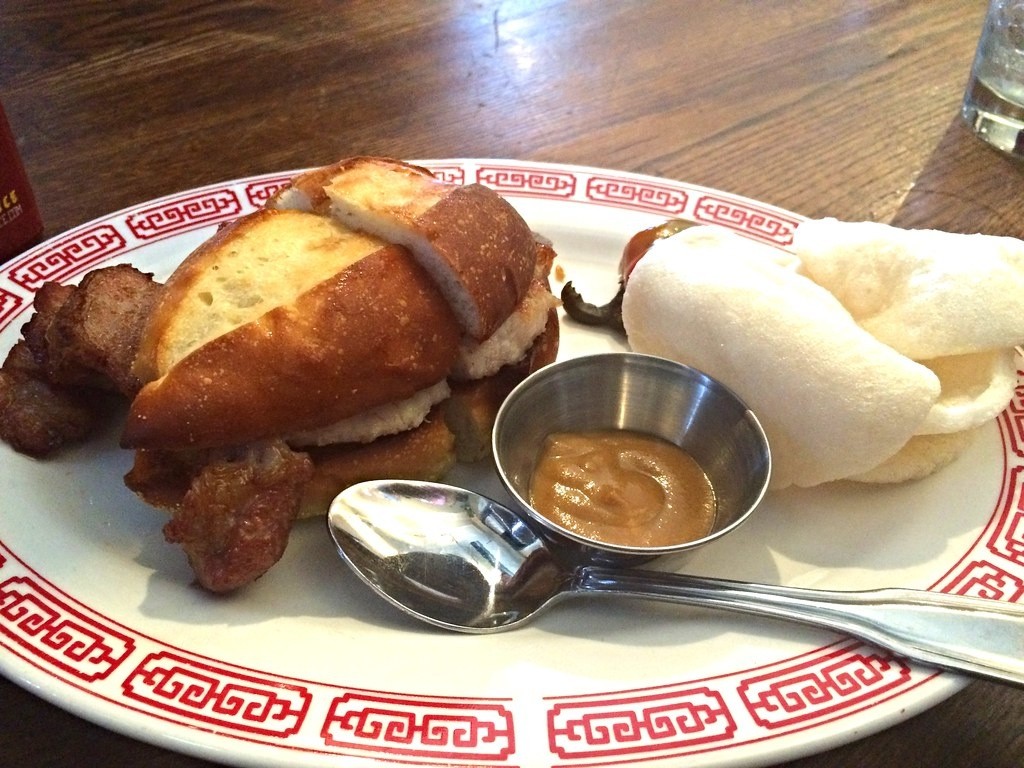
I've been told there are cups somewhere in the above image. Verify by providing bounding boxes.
[958,0,1024,160]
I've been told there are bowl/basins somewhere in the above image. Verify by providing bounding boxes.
[493,353,774,568]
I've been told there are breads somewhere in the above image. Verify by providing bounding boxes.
[116,158,562,521]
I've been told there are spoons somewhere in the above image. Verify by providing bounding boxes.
[326,478,1024,687]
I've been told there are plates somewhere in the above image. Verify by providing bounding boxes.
[0,153,1024,768]
